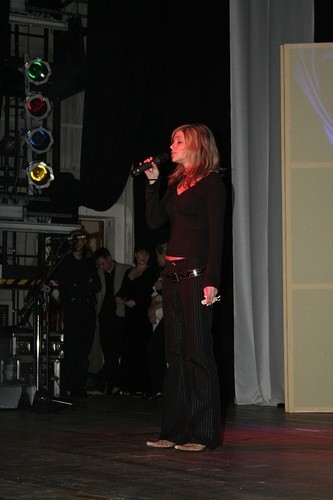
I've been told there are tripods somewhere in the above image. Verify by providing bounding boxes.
[19,239,90,410]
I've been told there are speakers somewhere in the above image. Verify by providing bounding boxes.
[0,384,32,409]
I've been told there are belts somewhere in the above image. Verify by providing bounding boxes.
[161,267,206,283]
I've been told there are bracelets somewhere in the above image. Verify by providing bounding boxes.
[148,178,158,181]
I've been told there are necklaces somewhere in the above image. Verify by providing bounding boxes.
[132,268,138,274]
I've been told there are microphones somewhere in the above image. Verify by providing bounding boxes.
[132,153,169,176]
[63,235,87,241]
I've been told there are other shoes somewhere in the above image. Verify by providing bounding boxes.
[103,387,164,401]
[174,443,210,452]
[145,439,176,448]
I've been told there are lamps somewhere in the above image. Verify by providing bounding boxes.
[24,58,56,190]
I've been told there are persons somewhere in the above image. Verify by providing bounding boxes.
[146,240,168,401]
[112,243,158,400]
[92,247,132,396]
[51,229,101,397]
[138,122,226,452]
